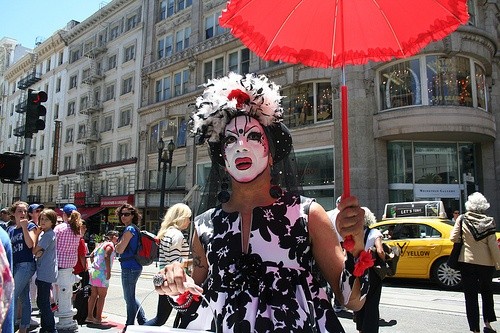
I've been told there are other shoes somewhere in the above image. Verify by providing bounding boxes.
[85,317,95,322]
[334,309,354,319]
[51,305,58,311]
[94,319,109,324]
[483,327,497,333]
[473,328,480,333]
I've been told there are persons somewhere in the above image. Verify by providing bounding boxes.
[324,192,500,333]
[153,71,376,333]
[0,201,192,333]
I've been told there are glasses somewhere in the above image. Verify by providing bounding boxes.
[31,209,43,214]
[118,212,131,217]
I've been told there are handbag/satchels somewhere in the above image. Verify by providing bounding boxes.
[448,214,466,266]
[344,250,369,276]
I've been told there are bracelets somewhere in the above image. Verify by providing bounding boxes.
[168,293,193,312]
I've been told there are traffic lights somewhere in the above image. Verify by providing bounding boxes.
[26,89,48,133]
[0,154,22,179]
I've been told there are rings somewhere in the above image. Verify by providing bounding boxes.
[153,275,167,287]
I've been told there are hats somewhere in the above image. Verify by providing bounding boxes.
[59,204,77,214]
[28,204,45,212]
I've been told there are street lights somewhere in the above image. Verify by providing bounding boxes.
[156,137,176,260]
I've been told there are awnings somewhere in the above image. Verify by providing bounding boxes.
[53,207,106,220]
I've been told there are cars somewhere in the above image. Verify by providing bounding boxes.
[367,218,500,290]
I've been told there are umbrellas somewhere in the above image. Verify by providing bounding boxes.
[217,0,470,254]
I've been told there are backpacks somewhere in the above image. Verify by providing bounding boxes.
[120,224,158,266]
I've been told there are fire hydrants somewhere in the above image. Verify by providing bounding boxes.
[53,267,82,333]
